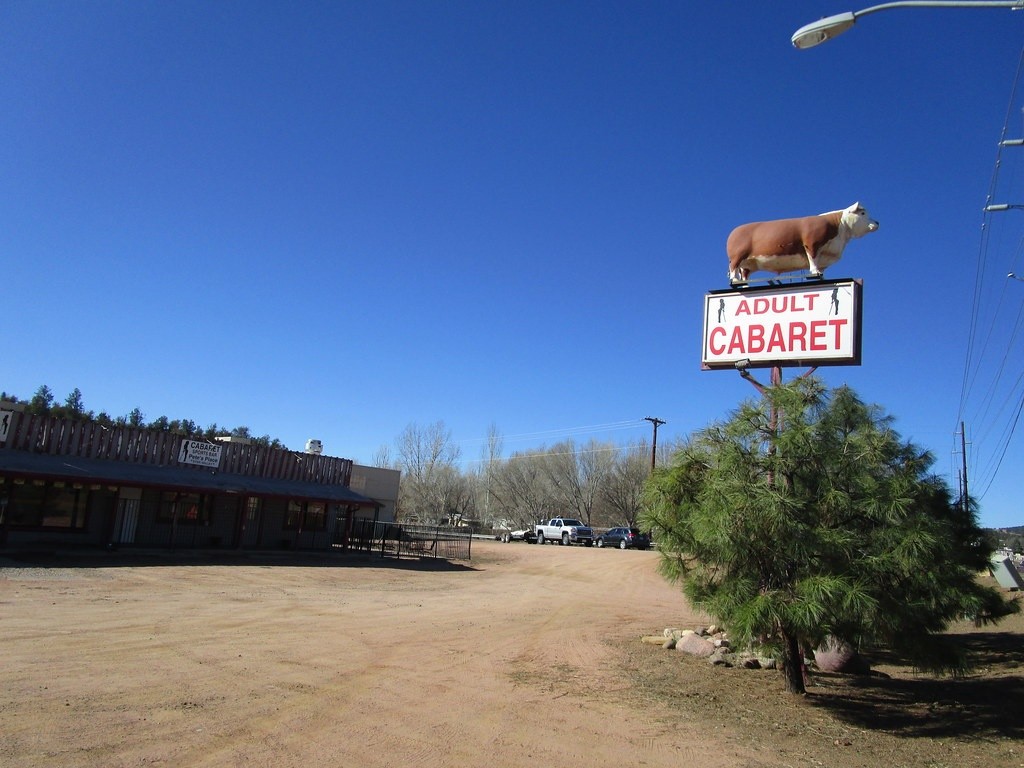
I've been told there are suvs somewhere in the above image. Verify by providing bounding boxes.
[596,527,650,550]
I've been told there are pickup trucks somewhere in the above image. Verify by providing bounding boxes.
[536,517,595,547]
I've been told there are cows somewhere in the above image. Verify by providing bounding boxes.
[727,201,879,288]
